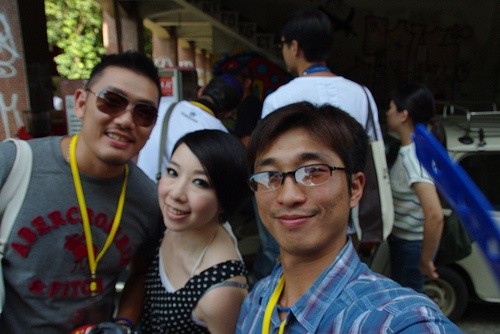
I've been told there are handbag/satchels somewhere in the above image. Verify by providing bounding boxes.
[352,85,395,243]
[438,212,472,261]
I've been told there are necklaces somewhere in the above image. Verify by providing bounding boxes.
[302,64,332,75]
[261,273,289,334]
[170,226,219,280]
[188,101,216,116]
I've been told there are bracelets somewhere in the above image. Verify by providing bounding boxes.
[113,317,136,328]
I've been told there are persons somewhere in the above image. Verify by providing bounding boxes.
[386,83,445,294]
[136,75,243,183]
[259,4,385,279]
[136,129,252,334]
[0,51,167,334]
[235,101,465,334]
[231,63,263,132]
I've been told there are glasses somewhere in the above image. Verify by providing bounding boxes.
[248,162,346,194]
[278,38,287,50]
[84,87,159,127]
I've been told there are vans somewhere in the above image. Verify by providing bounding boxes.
[371,109,500,325]
